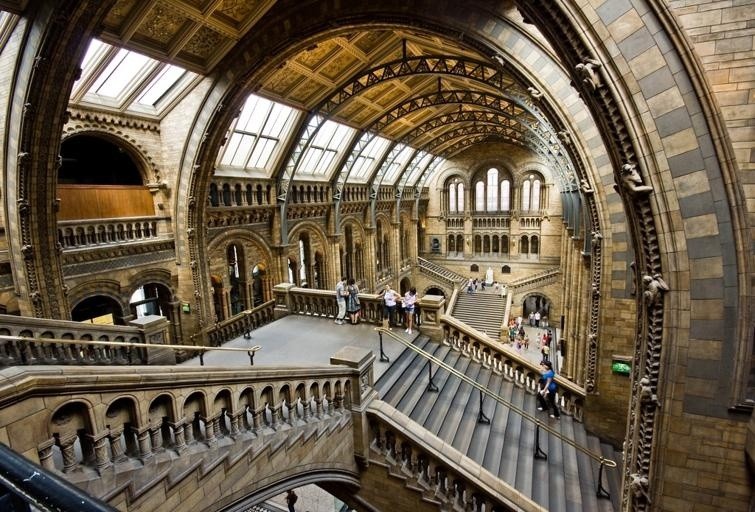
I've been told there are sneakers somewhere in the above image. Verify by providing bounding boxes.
[549,414,560,420]
[405,328,412,335]
[351,321,358,325]
[334,318,344,325]
[537,407,544,411]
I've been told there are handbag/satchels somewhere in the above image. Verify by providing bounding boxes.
[353,298,360,306]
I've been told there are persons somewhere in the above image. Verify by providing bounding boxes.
[465,276,507,300]
[508,311,552,367]
[401,287,419,335]
[536,360,561,419]
[284,489,298,512]
[374,283,402,330]
[345,278,360,325]
[334,276,348,324]
